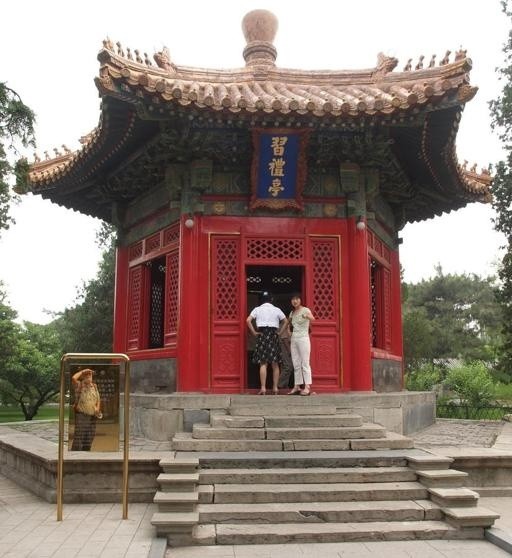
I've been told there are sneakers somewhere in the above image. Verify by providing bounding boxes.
[258,390,266,394]
[288,388,301,395]
[272,390,279,395]
[301,389,310,396]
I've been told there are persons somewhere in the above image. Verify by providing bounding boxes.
[246,291,289,395]
[287,293,315,396]
[278,308,294,389]
[71,369,101,451]
